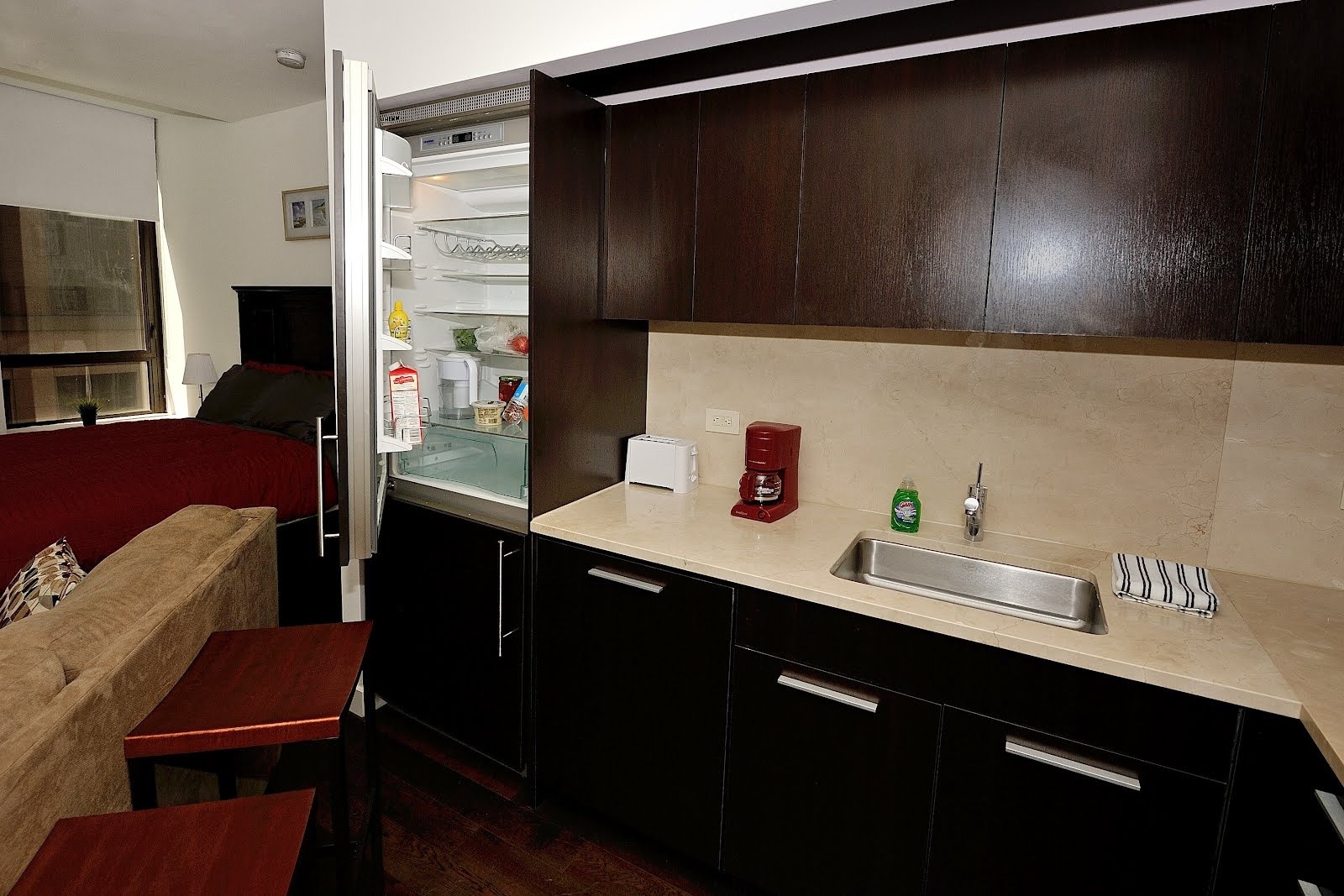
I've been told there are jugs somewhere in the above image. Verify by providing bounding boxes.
[435,352,482,419]
[738,468,782,502]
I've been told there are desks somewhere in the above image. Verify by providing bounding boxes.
[124,619,386,896]
[5,788,317,896]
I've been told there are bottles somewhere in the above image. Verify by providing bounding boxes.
[500,375,529,425]
[387,302,409,342]
[891,473,921,533]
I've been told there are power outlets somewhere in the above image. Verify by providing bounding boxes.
[705,408,745,436]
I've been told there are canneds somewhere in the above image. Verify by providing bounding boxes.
[498,376,522,406]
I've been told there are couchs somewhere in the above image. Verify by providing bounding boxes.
[0,503,283,896]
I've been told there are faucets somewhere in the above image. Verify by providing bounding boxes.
[962,484,988,543]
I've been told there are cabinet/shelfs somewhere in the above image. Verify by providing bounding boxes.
[363,523,534,770]
[612,0,1344,350]
[695,579,961,896]
[969,625,1344,896]
[506,536,736,887]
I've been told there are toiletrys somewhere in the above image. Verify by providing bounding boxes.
[890,471,921,533]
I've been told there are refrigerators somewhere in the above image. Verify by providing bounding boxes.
[311,50,604,748]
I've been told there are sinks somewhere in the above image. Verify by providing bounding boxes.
[829,530,1110,637]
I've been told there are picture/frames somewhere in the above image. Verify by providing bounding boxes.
[281,186,331,242]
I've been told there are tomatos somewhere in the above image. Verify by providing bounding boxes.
[512,336,528,354]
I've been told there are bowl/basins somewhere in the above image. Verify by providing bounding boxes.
[469,400,507,425]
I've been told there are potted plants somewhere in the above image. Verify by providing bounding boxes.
[71,394,104,427]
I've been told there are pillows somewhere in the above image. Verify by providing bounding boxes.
[0,538,87,640]
[195,365,343,440]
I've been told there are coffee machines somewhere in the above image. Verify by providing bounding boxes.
[730,421,801,523]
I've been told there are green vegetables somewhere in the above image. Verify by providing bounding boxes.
[453,329,477,350]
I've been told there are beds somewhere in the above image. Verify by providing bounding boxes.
[0,286,338,595]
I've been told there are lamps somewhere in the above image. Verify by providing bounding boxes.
[181,353,219,406]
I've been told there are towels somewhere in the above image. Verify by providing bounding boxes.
[1111,552,1220,620]
